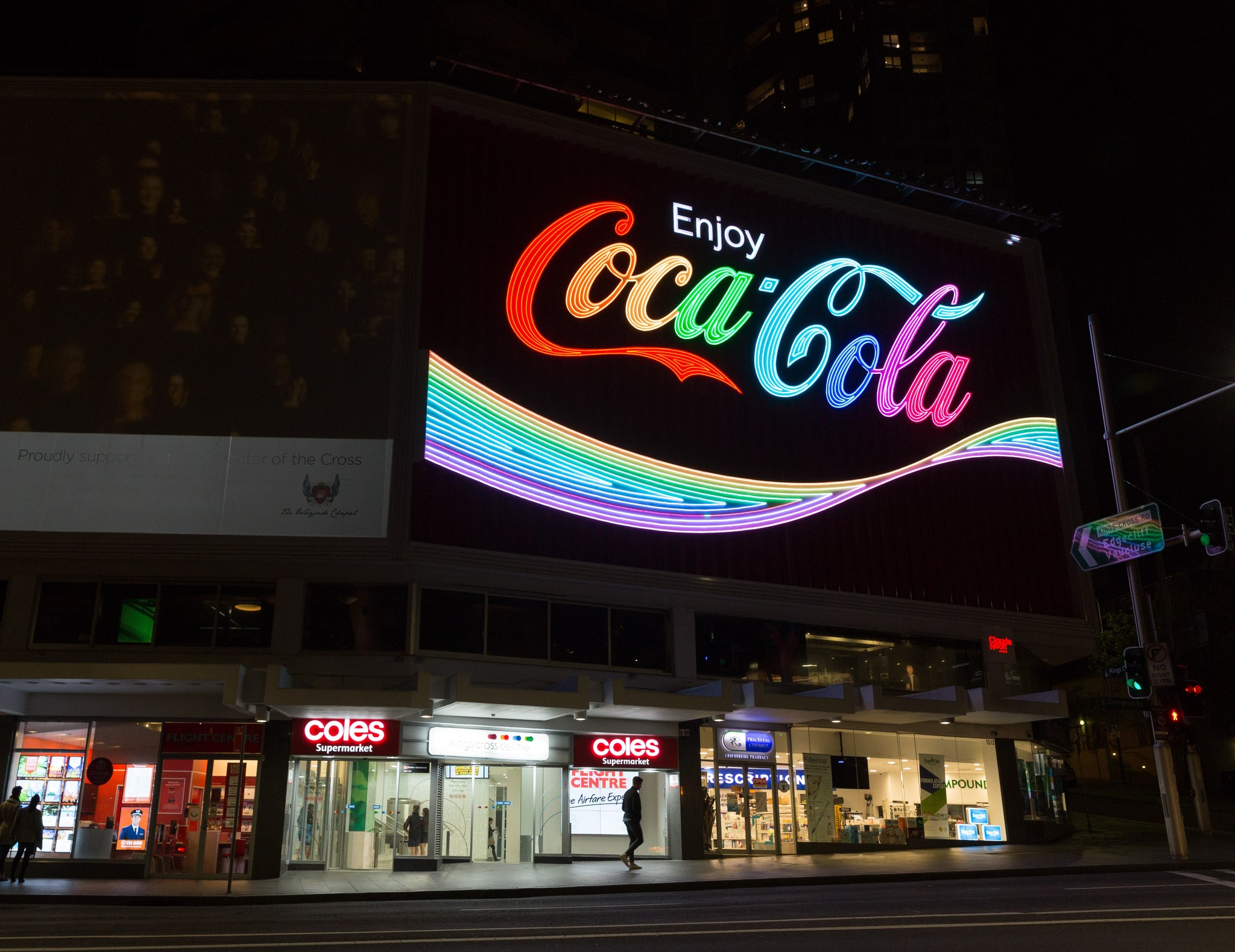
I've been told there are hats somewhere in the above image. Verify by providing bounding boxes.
[131,809,143,816]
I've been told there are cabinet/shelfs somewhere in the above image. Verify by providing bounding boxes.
[207,799,254,838]
[712,806,808,850]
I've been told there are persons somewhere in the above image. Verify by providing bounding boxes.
[621,776,644,870]
[119,809,145,840]
[404,805,423,855]
[9,794,44,883]
[488,818,501,861]
[0,786,22,882]
[297,803,319,860]
[419,808,429,855]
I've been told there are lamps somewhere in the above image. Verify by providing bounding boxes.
[255,704,270,722]
[939,718,952,725]
[573,710,587,720]
[419,700,433,718]
[712,714,725,722]
[830,717,842,723]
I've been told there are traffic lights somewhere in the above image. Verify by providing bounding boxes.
[1178,679,1206,719]
[1198,498,1227,558]
[1124,647,1153,698]
[1166,709,1185,724]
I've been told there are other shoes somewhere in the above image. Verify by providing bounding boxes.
[11,878,16,882]
[494,859,501,862]
[19,879,25,884]
[0,877,8,882]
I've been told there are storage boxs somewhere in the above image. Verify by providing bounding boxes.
[840,815,923,843]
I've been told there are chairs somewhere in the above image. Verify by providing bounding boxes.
[220,839,247,874]
[117,836,189,874]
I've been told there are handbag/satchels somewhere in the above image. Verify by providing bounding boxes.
[488,838,495,845]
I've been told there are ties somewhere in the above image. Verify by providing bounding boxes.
[134,828,137,835]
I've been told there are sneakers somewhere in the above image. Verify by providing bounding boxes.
[621,854,630,868]
[629,865,642,870]
